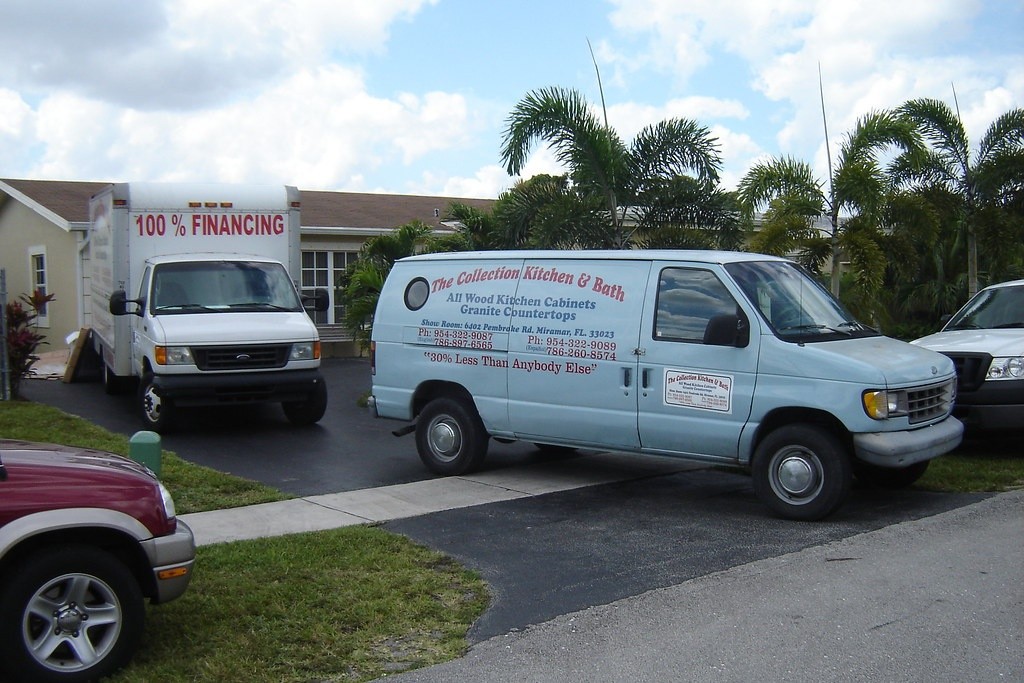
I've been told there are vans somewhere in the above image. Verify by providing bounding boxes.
[367,249,964,522]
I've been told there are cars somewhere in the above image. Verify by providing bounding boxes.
[0,438,195,683]
[908,280,1023,450]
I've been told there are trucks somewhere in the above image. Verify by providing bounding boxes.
[88,180,328,434]
